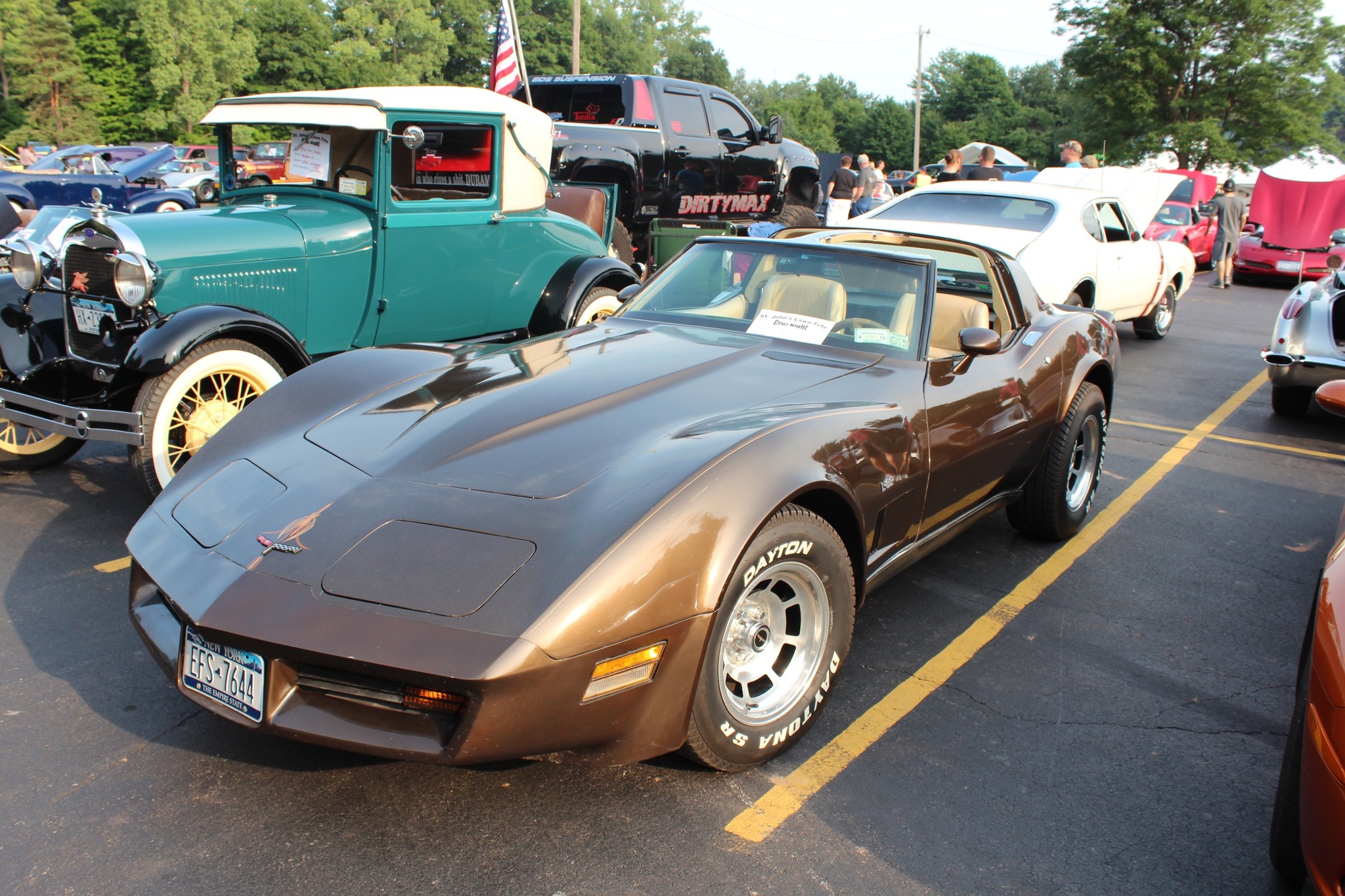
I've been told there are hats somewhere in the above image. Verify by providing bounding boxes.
[1058,140,1082,153]
[684,162,701,166]
[857,154,869,163]
[1223,179,1237,192]
[1079,155,1098,168]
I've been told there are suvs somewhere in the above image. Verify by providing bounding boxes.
[232,141,316,187]
[0,86,643,511]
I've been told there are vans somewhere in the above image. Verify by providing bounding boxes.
[173,146,250,169]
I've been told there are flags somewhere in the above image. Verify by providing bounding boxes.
[490,0,525,99]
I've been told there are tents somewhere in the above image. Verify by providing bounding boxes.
[938,141,1029,166]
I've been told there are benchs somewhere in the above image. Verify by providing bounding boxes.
[391,185,489,203]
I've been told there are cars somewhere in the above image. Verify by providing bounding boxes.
[0,145,200,214]
[25,147,148,177]
[138,159,220,202]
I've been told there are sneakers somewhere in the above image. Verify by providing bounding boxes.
[1208,279,1225,289]
[1224,276,1231,287]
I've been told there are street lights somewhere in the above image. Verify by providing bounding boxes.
[913,25,930,174]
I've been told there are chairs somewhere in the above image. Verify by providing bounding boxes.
[754,273,848,334]
[889,292,990,358]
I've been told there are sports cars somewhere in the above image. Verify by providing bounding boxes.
[124,225,1116,772]
[1142,170,1218,268]
[1233,168,1345,286]
[1259,227,1345,422]
[778,166,1196,341]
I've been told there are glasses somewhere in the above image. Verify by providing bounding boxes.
[1061,148,1071,152]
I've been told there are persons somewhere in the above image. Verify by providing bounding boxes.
[1057,141,1084,169]
[912,166,933,189]
[936,149,963,183]
[852,154,877,218]
[824,155,858,228]
[19,141,38,169]
[1079,155,1099,169]
[967,146,1004,181]
[873,160,888,183]
[1205,179,1247,289]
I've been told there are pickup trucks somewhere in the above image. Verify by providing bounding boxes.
[511,75,822,264]
